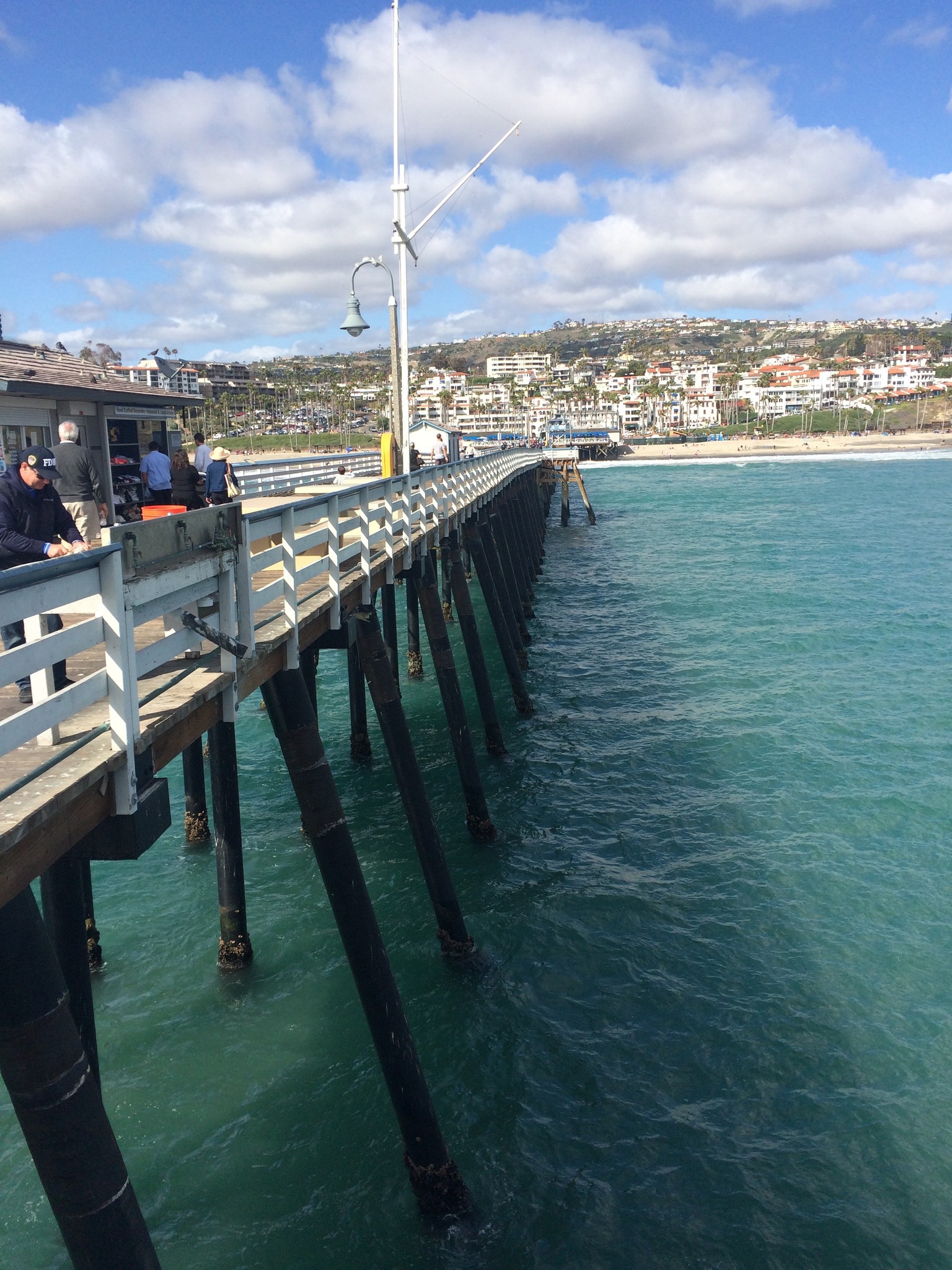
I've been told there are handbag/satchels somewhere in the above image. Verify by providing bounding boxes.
[415,450,425,465]
[224,462,242,498]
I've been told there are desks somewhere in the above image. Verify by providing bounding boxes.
[24,476,382,749]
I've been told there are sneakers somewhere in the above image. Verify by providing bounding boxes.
[19,687,33,703]
[53,676,75,690]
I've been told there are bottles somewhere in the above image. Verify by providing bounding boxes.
[99,510,107,525]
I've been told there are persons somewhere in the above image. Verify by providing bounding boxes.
[170,449,204,511]
[47,422,108,546]
[140,441,172,505]
[408,434,545,489]
[618,430,951,455]
[0,446,91,705]
[205,447,239,505]
[194,433,213,473]
[333,466,355,485]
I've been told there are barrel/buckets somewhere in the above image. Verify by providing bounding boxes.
[141,505,188,521]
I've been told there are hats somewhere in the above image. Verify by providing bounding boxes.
[469,443,472,445]
[209,447,230,460]
[22,446,62,480]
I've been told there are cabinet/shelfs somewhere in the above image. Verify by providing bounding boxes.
[109,443,157,523]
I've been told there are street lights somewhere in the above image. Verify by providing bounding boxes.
[339,258,403,475]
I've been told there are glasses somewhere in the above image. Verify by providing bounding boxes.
[32,468,46,480]
